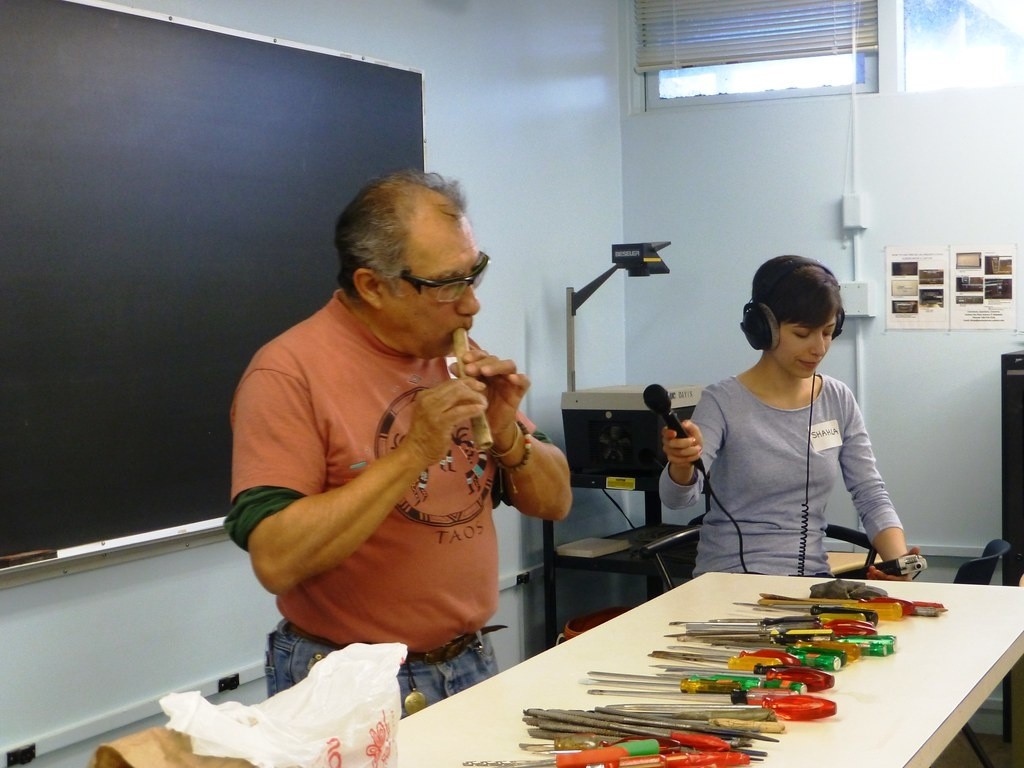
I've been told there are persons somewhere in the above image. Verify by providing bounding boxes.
[222,166,575,718]
[655,254,922,583]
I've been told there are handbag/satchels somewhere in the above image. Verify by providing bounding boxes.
[90,719,257,768]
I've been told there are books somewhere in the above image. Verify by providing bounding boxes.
[557,536,633,558]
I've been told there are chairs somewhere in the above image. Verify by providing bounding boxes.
[952,539,1010,768]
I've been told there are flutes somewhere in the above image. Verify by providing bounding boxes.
[452,327,494,449]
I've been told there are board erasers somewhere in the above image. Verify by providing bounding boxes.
[0,549,57,569]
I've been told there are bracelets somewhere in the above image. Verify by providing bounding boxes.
[487,420,520,455]
[492,420,533,494]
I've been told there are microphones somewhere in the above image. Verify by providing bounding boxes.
[643,384,705,475]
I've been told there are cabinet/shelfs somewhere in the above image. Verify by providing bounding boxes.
[543,469,711,652]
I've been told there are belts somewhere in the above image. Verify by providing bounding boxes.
[288,623,508,664]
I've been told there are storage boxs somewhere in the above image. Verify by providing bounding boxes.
[561,385,704,475]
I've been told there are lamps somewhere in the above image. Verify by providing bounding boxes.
[566,241,671,391]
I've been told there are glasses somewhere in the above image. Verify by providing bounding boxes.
[400,252,491,302]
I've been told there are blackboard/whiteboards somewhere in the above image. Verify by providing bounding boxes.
[0,0,426,574]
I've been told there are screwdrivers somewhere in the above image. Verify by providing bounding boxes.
[462,592,915,768]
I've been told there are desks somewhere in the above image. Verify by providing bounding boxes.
[398,572,1024,768]
[825,551,886,579]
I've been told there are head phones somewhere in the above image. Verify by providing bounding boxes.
[740,257,845,351]
